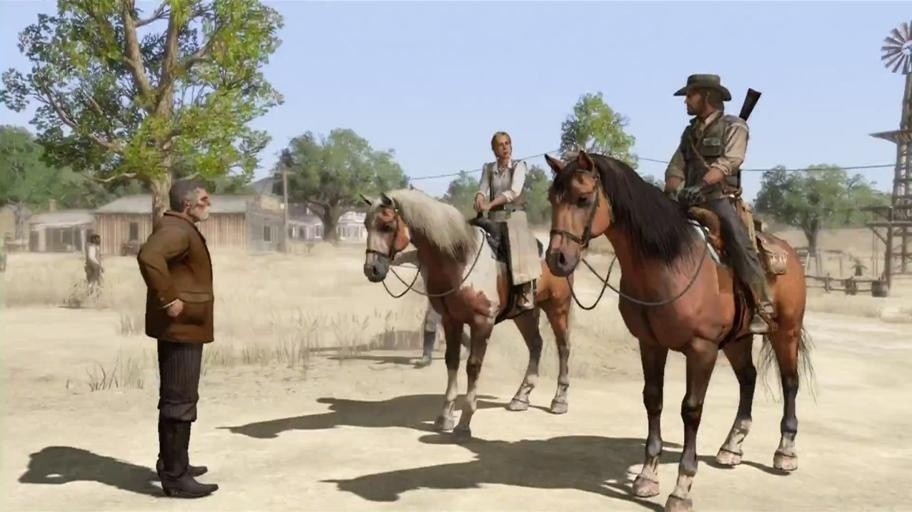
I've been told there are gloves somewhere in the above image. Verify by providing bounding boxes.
[678,181,704,201]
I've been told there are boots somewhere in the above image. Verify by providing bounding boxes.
[516,281,535,310]
[414,332,435,365]
[459,333,471,360]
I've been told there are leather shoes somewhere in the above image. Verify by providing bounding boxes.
[162,474,217,496]
[750,313,773,334]
[156,461,207,479]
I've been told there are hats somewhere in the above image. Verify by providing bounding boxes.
[673,73,731,101]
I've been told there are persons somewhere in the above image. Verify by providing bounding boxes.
[665,73,773,334]
[474,133,537,311]
[85,235,104,299]
[136,177,218,499]
[397,249,471,368]
